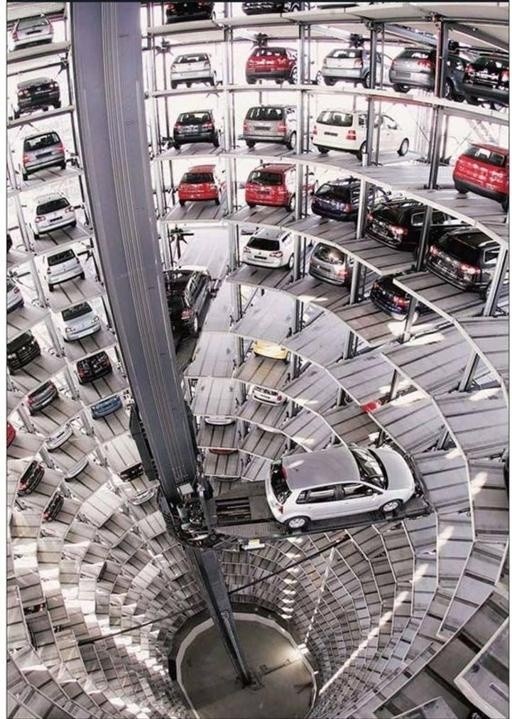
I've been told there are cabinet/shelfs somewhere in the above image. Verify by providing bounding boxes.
[6,1,509,719]
